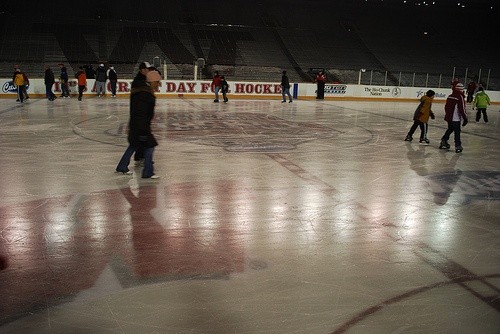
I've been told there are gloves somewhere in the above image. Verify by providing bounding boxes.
[462,118,468,126]
[431,114,435,119]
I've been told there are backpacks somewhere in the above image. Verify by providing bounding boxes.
[109,69,117,82]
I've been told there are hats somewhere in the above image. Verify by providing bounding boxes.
[145,70,161,82]
[139,61,154,69]
[455,83,466,89]
[426,90,436,97]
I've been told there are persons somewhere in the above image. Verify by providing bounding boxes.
[78,69,87,101]
[440,80,469,150]
[315,71,326,83]
[109,67,117,97]
[451,78,459,93]
[280,70,292,103]
[58,63,68,98]
[116,61,160,179]
[77,63,120,78]
[95,64,107,96]
[44,65,57,101]
[472,86,491,124]
[213,71,228,102]
[466,77,477,103]
[406,90,435,142]
[12,65,30,103]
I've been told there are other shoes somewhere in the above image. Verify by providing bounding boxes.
[214,98,220,102]
[16,98,20,102]
[110,95,117,98]
[59,96,70,99]
[281,101,286,103]
[484,121,489,124]
[439,141,451,149]
[454,145,463,153]
[133,159,154,167]
[151,175,160,179]
[419,136,430,145]
[223,98,228,102]
[404,133,413,142]
[24,97,29,101]
[289,101,292,103]
[115,169,133,176]
